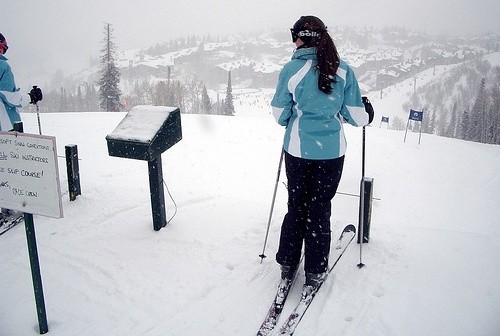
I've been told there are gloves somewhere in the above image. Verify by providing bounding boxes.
[28,87,43,105]
[362,95,375,125]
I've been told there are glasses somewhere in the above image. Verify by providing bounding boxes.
[290,29,322,43]
[0,40,9,53]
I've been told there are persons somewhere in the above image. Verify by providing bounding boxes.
[0,33,43,133]
[270,15,374,289]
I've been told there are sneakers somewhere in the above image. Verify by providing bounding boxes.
[1,207,23,223]
[305,268,330,288]
[279,263,299,277]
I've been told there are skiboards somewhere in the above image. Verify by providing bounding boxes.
[256,224,356,336]
[0,211,24,235]
